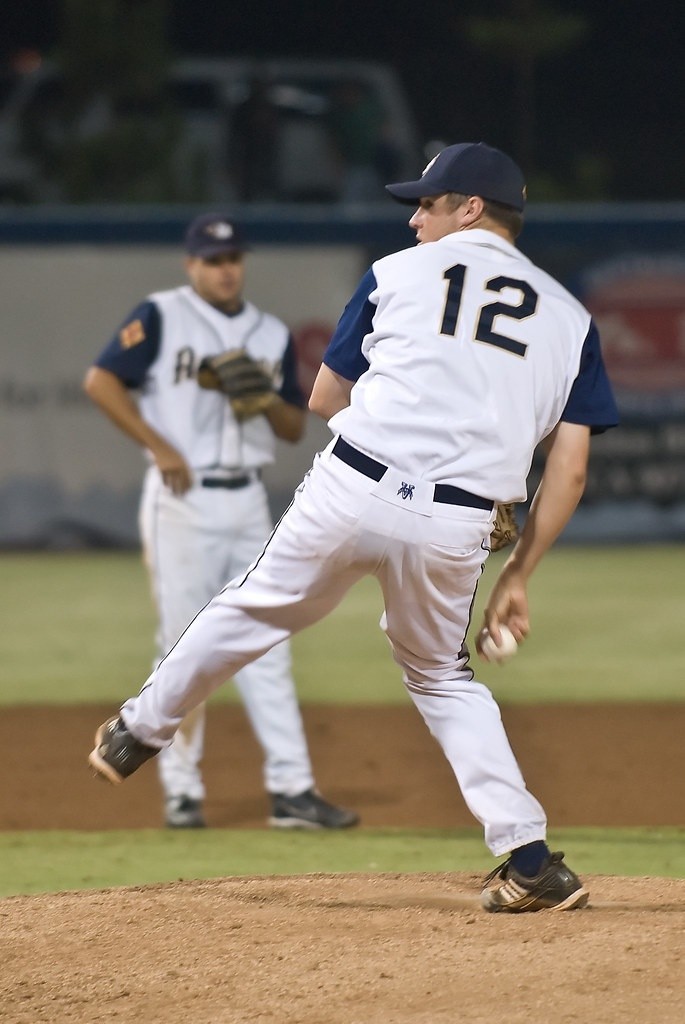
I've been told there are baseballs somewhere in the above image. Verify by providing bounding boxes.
[474,621,519,666]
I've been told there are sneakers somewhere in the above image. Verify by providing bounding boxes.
[88,713,160,786]
[479,850,588,916]
[267,790,358,829]
[165,796,206,829]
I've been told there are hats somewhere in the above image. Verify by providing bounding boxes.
[188,213,244,258]
[385,143,527,211]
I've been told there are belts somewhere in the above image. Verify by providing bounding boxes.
[332,437,495,511]
[202,468,262,490]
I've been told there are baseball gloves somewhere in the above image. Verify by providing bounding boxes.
[193,342,282,423]
[485,495,526,556]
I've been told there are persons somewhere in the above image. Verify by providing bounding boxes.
[81,211,360,832]
[87,141,617,914]
